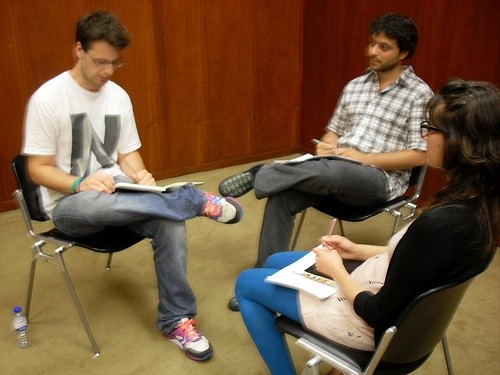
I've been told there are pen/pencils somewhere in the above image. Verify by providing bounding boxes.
[322,219,337,246]
[313,139,337,151]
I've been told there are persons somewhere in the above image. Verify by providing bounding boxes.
[19,8,244,361]
[219,12,436,312]
[234,79,500,375]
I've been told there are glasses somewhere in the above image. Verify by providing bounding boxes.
[420,120,448,137]
[86,51,125,69]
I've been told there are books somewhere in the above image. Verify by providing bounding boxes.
[112,181,205,195]
[263,243,366,301]
[273,153,363,166]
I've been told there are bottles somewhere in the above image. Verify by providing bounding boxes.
[12,306,31,348]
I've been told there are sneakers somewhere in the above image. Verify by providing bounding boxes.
[161,317,213,361]
[195,187,243,224]
[219,163,268,198]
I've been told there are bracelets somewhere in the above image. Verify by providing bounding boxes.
[72,177,84,193]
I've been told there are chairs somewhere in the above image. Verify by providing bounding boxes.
[11,155,148,357]
[275,244,497,375]
[290,163,428,251]
